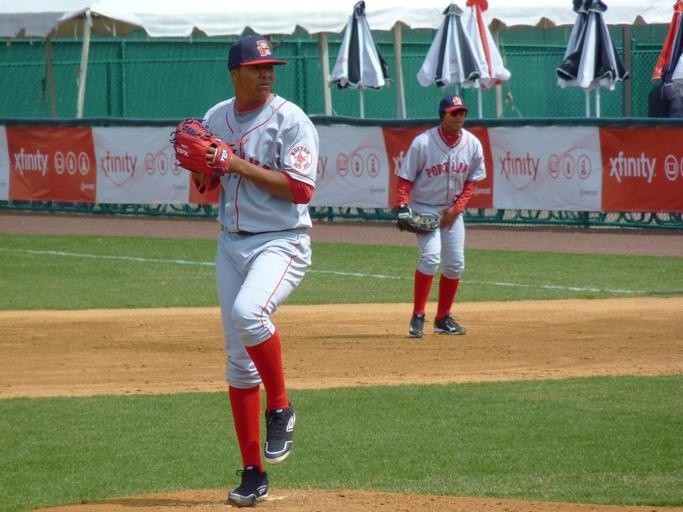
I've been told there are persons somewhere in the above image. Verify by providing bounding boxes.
[171,34,321,509]
[660,76,682,120]
[646,63,670,118]
[393,92,486,339]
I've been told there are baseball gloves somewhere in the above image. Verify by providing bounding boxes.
[399,210,441,233]
[172,118,232,176]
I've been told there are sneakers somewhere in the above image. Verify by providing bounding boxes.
[264,401,296,464]
[434,316,465,335]
[408,313,426,338]
[228,465,269,507]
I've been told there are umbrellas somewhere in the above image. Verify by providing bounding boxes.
[460,0,511,119]
[555,0,632,119]
[326,0,392,117]
[415,0,493,97]
[553,1,592,119]
[649,0,683,81]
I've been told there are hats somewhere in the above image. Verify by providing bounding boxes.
[439,95,467,117]
[228,35,287,71]
